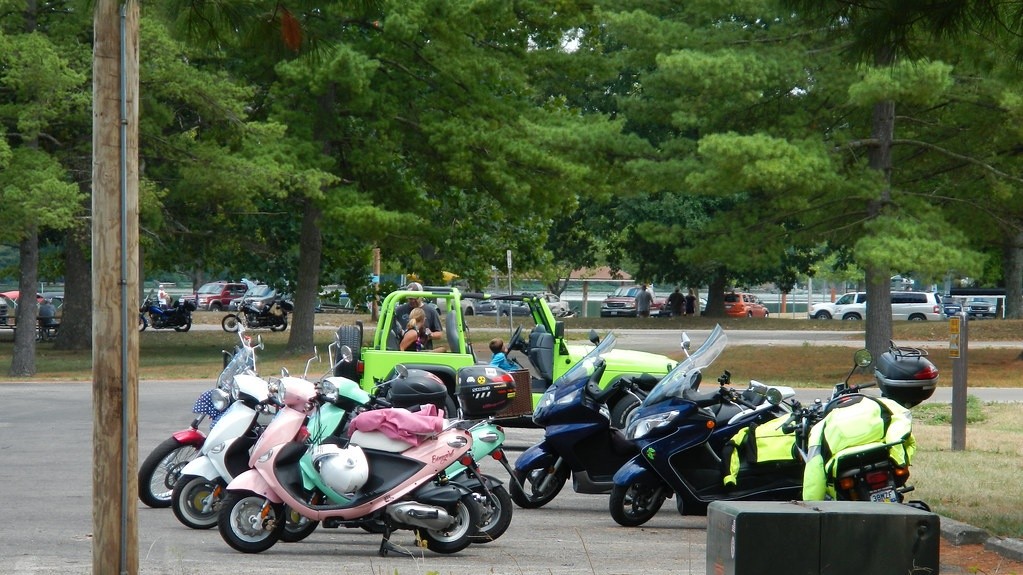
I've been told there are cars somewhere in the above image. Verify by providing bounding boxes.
[659,295,707,316]
[229,285,287,312]
[723,292,770,318]
[432,290,571,317]
[942,297,997,320]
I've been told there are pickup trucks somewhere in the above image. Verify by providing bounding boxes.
[599,284,668,318]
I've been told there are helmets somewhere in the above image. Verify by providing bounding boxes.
[312,444,370,493]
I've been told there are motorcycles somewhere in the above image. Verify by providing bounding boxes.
[509,321,933,528]
[222,290,294,332]
[138,289,198,331]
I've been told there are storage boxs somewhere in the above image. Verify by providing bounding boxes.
[387,369,447,409]
[874,351,940,402]
[185,300,197,311]
[457,365,517,417]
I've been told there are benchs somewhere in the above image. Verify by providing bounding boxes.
[0,315,61,342]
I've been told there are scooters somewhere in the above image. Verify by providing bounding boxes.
[137,321,530,558]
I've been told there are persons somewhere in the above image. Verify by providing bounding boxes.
[635,285,654,318]
[665,289,697,316]
[39,300,58,336]
[396,282,446,352]
[489,338,518,370]
[157,285,167,306]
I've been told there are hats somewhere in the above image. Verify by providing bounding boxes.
[159,285,164,287]
[641,285,647,289]
[674,286,680,290]
[405,282,423,299]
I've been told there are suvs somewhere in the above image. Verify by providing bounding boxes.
[808,291,943,322]
[333,283,679,412]
[179,277,249,312]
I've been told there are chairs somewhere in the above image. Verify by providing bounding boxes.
[446,310,478,364]
[379,329,400,351]
